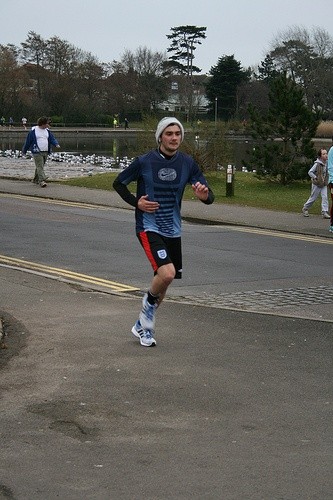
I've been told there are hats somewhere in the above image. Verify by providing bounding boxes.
[155,117,184,147]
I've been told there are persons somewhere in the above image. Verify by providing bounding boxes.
[8,117,16,130]
[328,137,333,233]
[1,116,8,130]
[302,147,331,219]
[22,116,28,130]
[21,116,61,187]
[47,118,52,129]
[112,116,215,346]
[113,118,118,129]
[124,118,130,130]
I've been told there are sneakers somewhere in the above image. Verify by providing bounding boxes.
[302,207,309,216]
[131,320,156,346]
[329,226,333,233]
[321,211,330,218]
[139,293,158,329]
[39,181,47,186]
